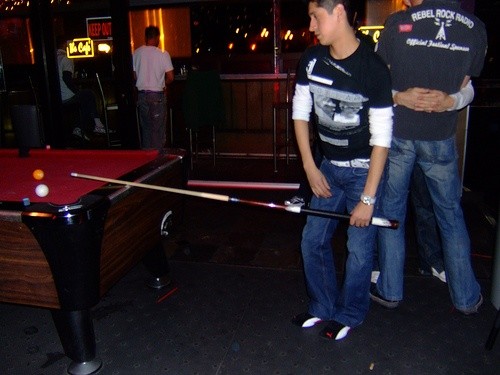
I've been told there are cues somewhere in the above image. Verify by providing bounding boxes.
[70,172,400,230]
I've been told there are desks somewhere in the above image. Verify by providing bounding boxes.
[0,145,188,375]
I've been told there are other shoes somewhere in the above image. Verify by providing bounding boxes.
[457,292,483,315]
[430,261,447,284]
[291,313,324,328]
[93,127,112,134]
[73,127,90,141]
[368,282,400,308]
[319,321,364,342]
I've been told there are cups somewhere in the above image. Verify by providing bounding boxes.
[179,64,188,80]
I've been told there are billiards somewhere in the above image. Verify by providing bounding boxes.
[32,169,44,181]
[35,184,49,197]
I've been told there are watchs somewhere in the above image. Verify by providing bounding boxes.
[360,194,376,205]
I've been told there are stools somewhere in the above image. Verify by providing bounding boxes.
[92,70,314,173]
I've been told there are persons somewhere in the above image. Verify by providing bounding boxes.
[132,25,175,148]
[288,0,397,339]
[57,35,115,141]
[369,0,487,314]
